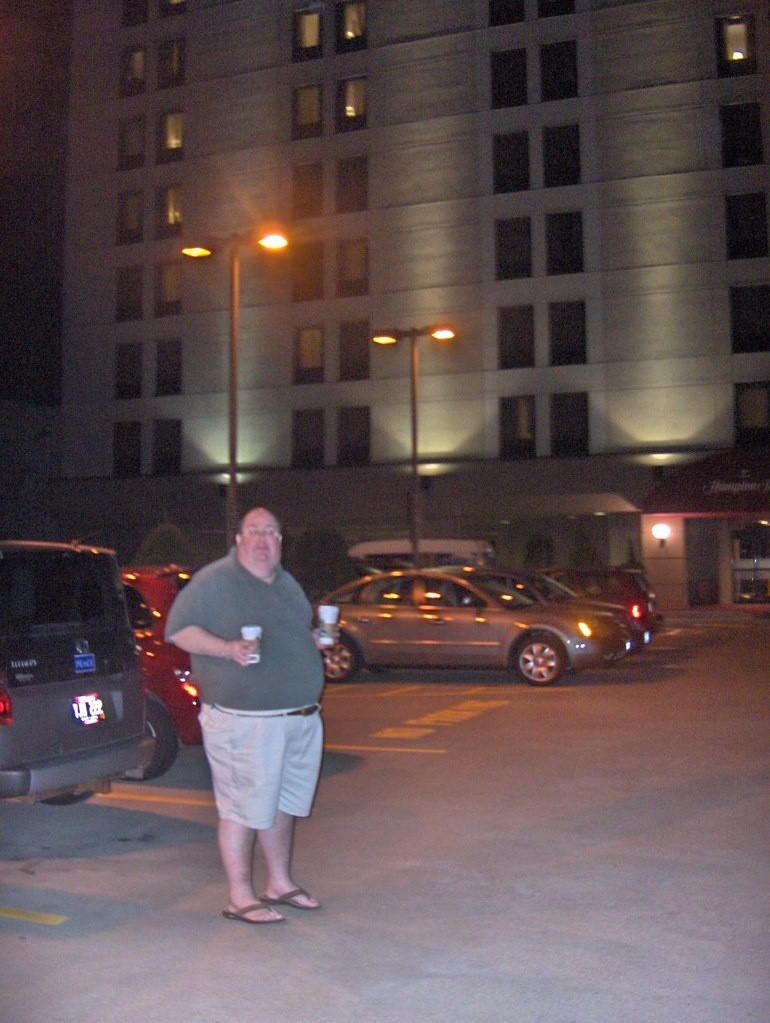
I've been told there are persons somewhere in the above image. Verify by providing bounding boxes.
[165,507,339,923]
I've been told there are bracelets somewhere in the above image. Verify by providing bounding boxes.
[226,641,232,662]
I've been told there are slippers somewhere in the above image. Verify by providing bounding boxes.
[259,888,319,909]
[222,902,285,923]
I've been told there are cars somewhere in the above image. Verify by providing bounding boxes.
[311,568,632,686]
[114,564,204,783]
[483,563,664,660]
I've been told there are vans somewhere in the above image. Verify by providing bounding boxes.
[0,539,155,807]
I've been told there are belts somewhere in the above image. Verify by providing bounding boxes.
[212,705,318,717]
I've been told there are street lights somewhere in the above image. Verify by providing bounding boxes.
[372,324,458,567]
[180,230,290,555]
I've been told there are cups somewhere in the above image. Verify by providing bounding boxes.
[241,625,262,663]
[318,606,340,645]
